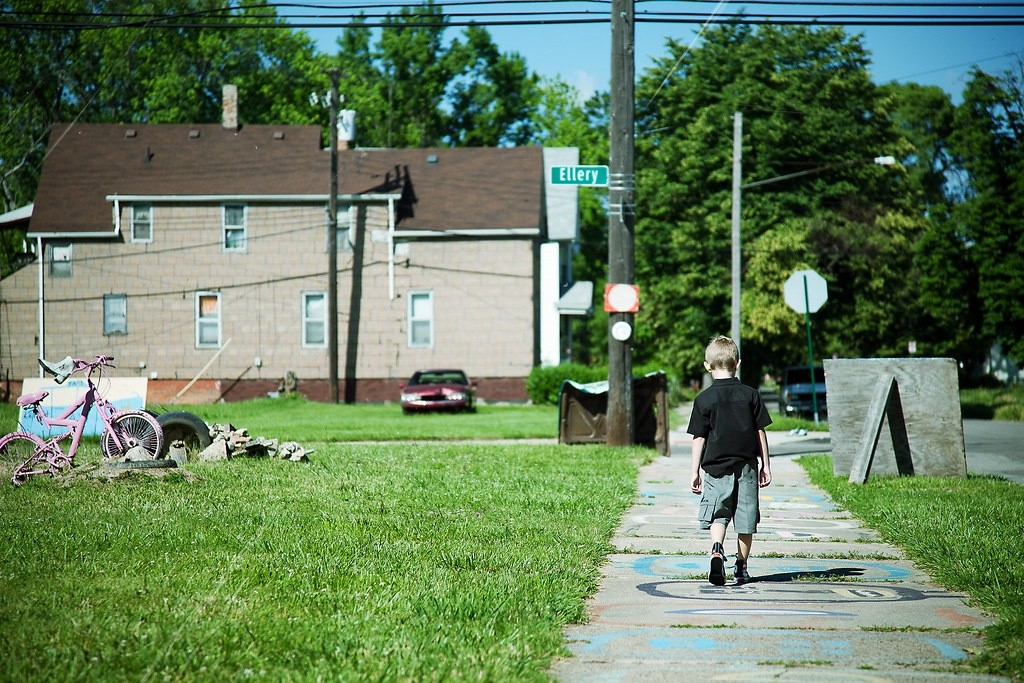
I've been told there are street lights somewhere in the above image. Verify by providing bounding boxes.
[725,108,901,388]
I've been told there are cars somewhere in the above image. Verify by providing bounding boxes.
[399,369,479,416]
[774,364,828,422]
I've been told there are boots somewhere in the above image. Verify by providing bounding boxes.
[38,356,75,384]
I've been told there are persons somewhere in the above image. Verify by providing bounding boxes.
[690,334,773,585]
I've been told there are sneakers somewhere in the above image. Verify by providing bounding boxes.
[709,542,727,586]
[734,561,750,584]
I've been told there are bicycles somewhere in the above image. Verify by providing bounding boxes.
[0,353,165,489]
